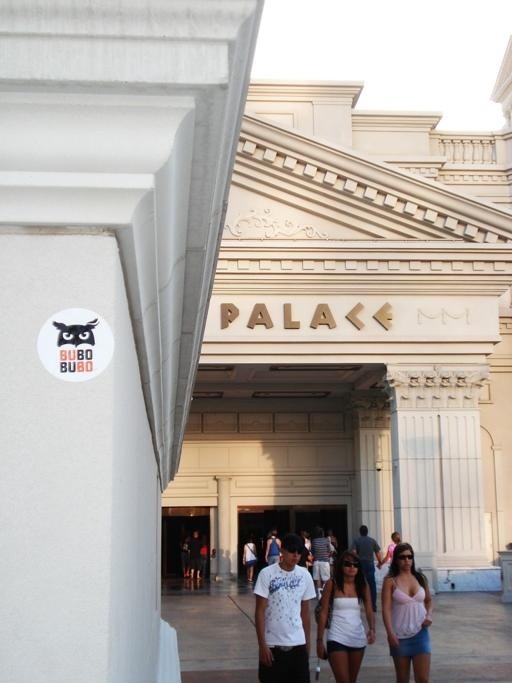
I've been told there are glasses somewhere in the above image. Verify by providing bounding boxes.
[343,561,360,568]
[399,555,414,560]
[287,548,304,555]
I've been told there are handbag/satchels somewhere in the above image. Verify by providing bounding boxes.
[315,600,333,629]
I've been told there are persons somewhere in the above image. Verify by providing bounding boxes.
[250,533,316,682]
[316,548,377,683]
[180,521,401,612]
[379,542,435,683]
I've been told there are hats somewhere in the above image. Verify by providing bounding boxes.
[280,533,309,555]
